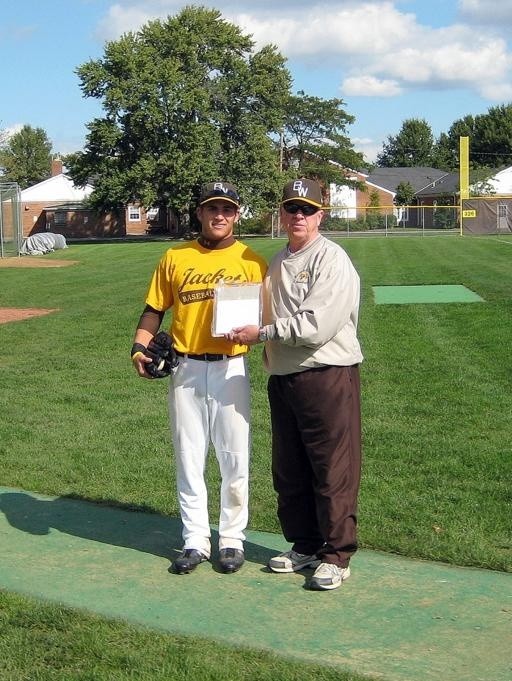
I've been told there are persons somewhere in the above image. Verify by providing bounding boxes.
[130,181,269,576]
[220,178,363,593]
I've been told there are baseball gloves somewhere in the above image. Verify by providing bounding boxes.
[144,331,179,377]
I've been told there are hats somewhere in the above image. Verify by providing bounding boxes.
[282,179,322,209]
[200,181,241,210]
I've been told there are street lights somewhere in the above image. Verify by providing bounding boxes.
[275,127,283,238]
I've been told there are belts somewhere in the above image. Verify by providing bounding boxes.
[174,350,230,362]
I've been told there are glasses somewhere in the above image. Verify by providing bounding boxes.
[283,202,318,216]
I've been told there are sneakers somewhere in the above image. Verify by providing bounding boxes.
[308,563,351,592]
[267,549,323,574]
[219,549,245,575]
[174,549,208,575]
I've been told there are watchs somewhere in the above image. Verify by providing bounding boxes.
[258,325,267,343]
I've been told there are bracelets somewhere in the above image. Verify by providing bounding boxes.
[130,343,147,364]
